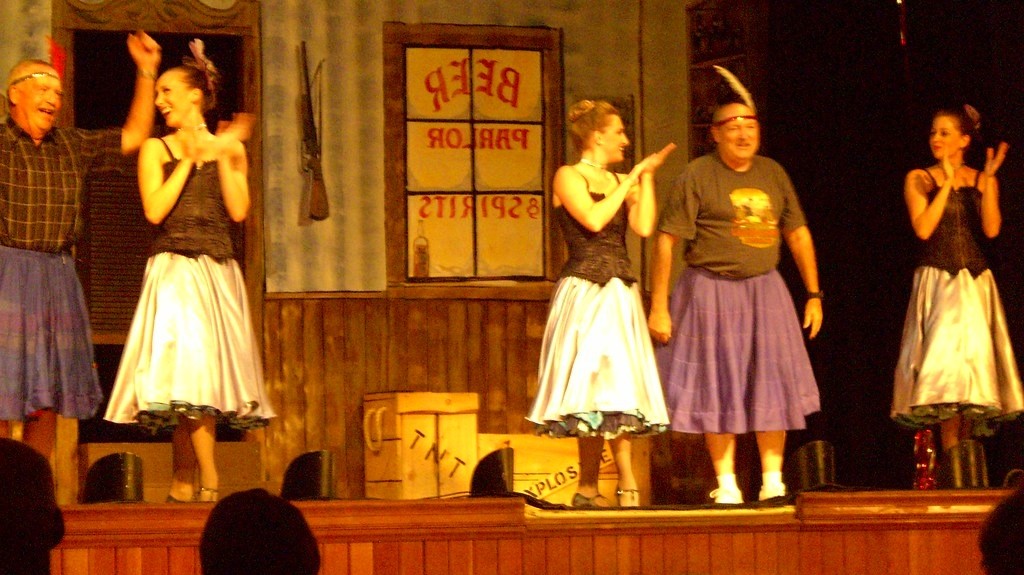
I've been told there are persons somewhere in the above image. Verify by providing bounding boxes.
[103,65,279,504]
[0,29,161,461]
[978,488,1024,575]
[890,103,1024,487]
[198,489,320,575]
[525,99,676,508]
[648,103,823,504]
[0,439,64,575]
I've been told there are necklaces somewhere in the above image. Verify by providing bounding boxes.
[937,161,964,171]
[177,123,208,133]
[579,159,608,170]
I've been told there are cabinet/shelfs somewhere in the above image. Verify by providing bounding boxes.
[363,392,479,501]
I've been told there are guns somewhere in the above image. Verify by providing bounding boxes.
[297,42,331,222]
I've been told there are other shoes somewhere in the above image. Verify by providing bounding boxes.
[572,485,640,508]
[165,487,218,501]
[708,486,744,504]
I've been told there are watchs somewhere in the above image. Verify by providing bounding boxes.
[807,290,824,299]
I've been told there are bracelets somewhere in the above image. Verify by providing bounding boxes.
[136,68,158,81]
